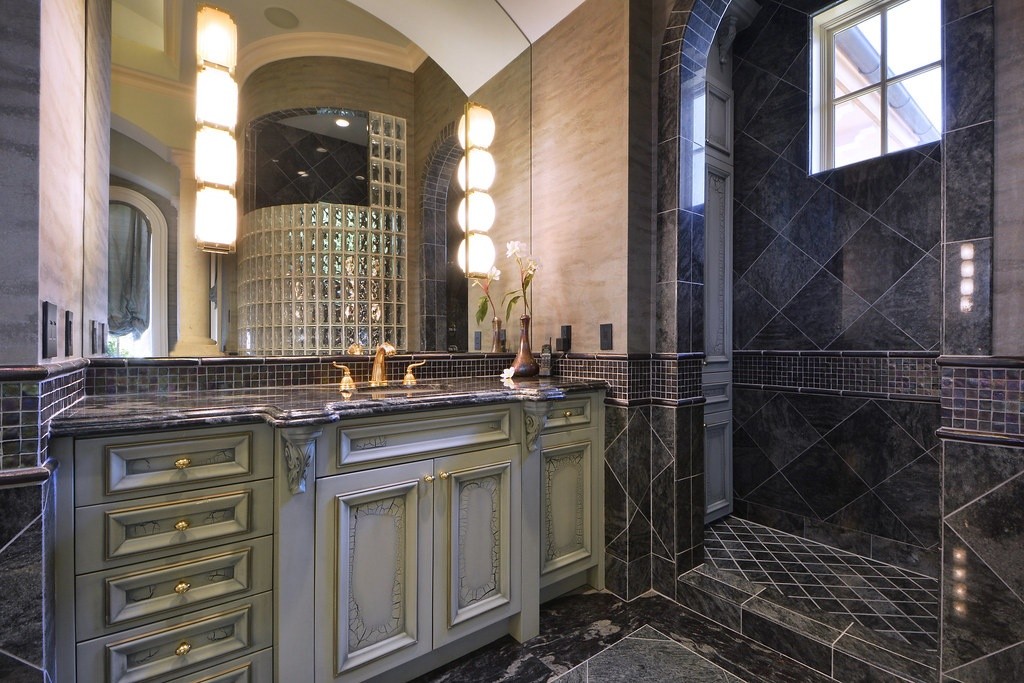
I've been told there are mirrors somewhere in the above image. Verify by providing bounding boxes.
[83,1,533,359]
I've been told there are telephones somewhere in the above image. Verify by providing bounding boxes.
[538,337,553,376]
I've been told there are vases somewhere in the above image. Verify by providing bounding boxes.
[506,314,539,377]
[491,317,505,354]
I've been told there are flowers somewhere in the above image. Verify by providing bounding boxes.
[463,266,501,326]
[499,239,540,321]
[499,366,516,390]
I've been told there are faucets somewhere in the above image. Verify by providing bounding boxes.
[347,343,364,355]
[367,342,396,387]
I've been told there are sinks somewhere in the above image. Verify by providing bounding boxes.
[347,385,445,394]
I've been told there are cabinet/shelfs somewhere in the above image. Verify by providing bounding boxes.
[47,391,609,683]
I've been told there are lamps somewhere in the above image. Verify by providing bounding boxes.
[193,3,242,253]
[453,102,499,280]
